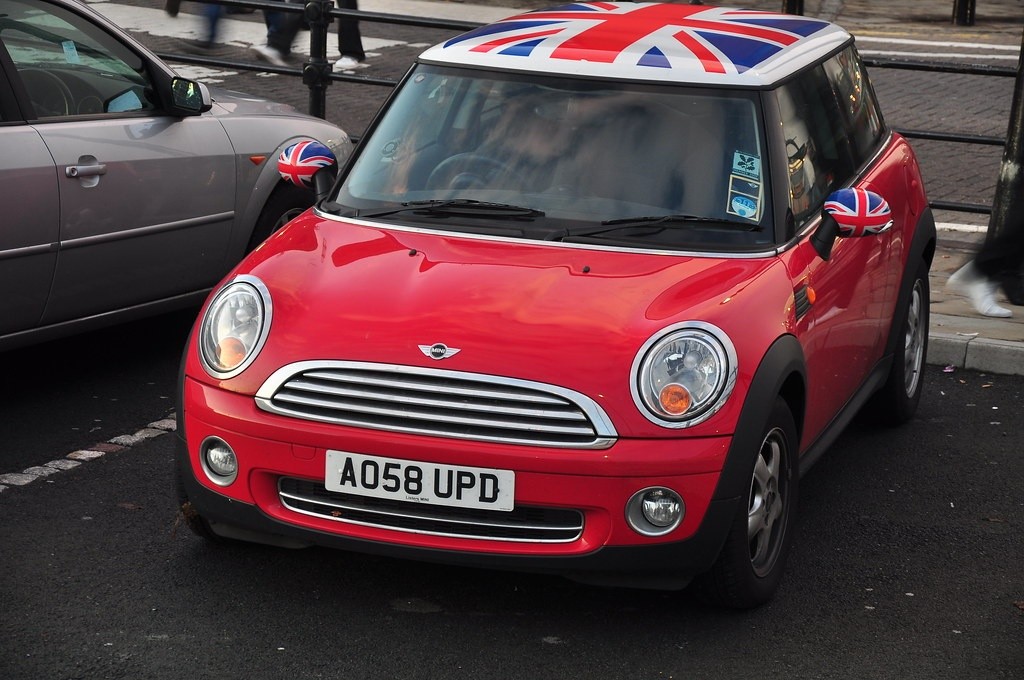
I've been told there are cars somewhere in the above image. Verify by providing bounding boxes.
[0,0,357,353]
[173,1,938,617]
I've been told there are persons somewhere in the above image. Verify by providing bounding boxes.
[250,0,365,72]
[177,0,304,61]
[943,212,1024,317]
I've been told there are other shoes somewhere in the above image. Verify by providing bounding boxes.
[335,56,359,69]
[946,262,1012,318]
[249,46,288,68]
